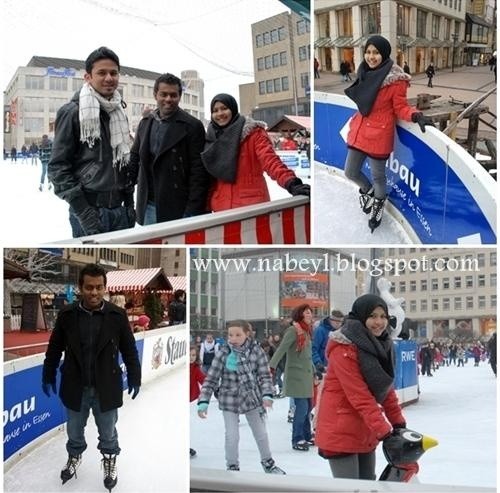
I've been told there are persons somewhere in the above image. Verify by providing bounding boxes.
[189,345,210,458]
[345,34,437,230]
[314,55,353,82]
[425,61,435,88]
[195,318,285,474]
[189,309,348,431]
[124,290,186,333]
[41,262,142,489]
[272,135,310,180]
[409,330,496,378]
[312,294,406,480]
[128,73,206,226]
[403,61,411,88]
[201,93,310,211]
[4,133,53,192]
[267,304,319,452]
[488,56,495,72]
[44,46,135,237]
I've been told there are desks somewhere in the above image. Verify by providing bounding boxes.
[405,95,491,161]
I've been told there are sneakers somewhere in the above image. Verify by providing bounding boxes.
[359,184,375,214]
[61,453,81,480]
[101,453,117,489]
[261,458,286,474]
[293,439,309,451]
[369,197,387,229]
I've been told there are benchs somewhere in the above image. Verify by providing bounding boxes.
[433,124,496,163]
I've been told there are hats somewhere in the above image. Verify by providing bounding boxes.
[330,311,345,322]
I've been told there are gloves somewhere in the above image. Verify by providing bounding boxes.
[42,382,57,398]
[128,385,139,399]
[412,112,436,133]
[286,177,310,196]
[72,204,107,236]
